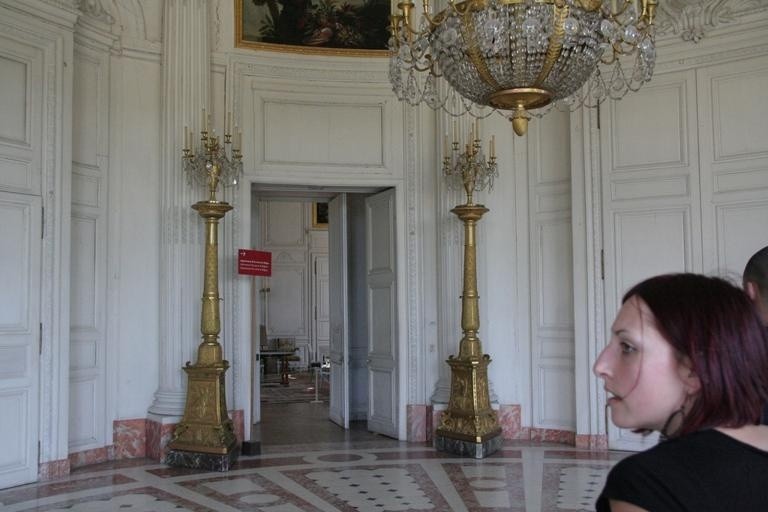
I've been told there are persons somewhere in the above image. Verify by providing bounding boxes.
[744,246,768,331]
[593,274,768,512]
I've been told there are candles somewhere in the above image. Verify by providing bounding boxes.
[438,118,499,163]
[184,105,244,160]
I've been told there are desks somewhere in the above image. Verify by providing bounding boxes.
[259,350,294,388]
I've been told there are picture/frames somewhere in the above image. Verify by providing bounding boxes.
[233,1,394,59]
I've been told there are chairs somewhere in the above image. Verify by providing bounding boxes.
[273,336,300,376]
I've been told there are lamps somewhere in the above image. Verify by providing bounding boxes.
[382,0,661,136]
